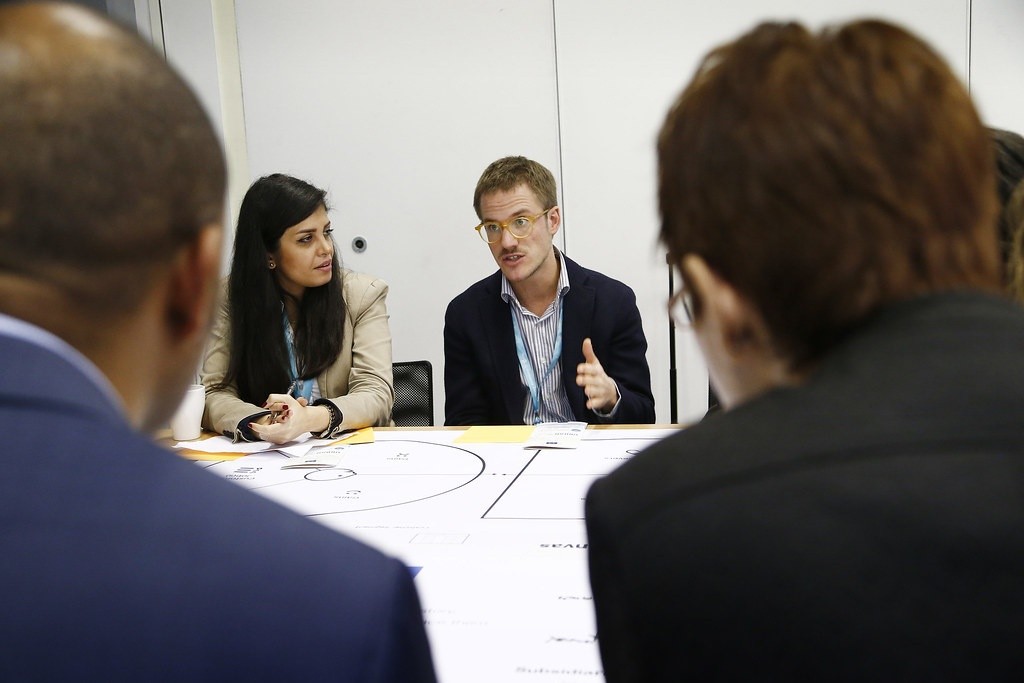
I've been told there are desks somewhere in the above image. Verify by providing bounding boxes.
[150,424,688,683]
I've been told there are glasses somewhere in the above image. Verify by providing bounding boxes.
[475,208,551,244]
[664,284,700,332]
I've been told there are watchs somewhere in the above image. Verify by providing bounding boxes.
[311,404,335,439]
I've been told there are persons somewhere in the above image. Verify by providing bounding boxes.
[0,0,437,683]
[200,173,394,445]
[444,156,655,425]
[585,17,1024,683]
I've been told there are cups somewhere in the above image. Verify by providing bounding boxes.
[168,384,206,441]
